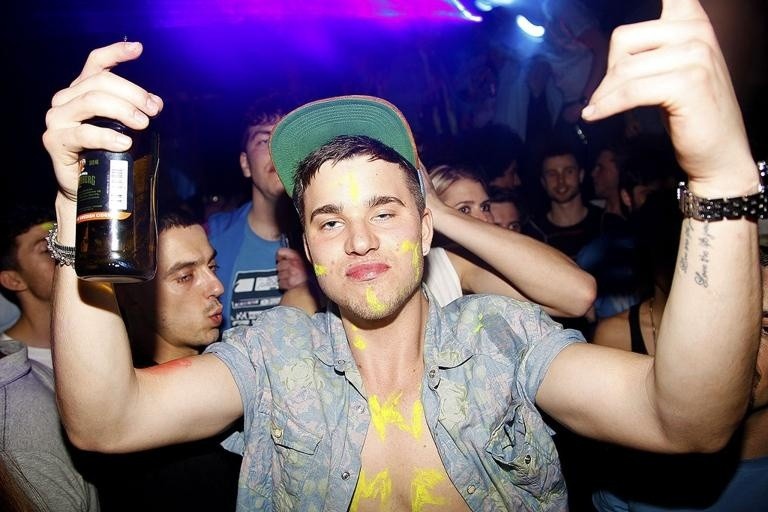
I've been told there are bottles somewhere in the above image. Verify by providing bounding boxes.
[75,31,158,285]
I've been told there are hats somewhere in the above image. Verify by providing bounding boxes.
[266,93,426,204]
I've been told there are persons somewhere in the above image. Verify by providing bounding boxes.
[3,2,766,512]
